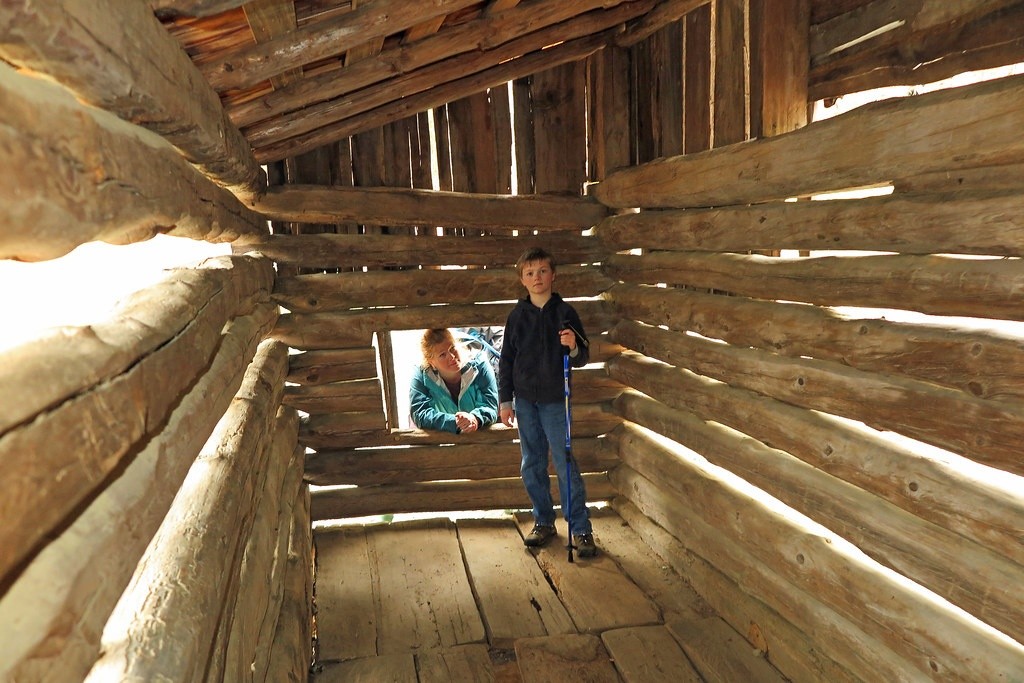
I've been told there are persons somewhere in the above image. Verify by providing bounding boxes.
[495,245,597,559]
[409,327,498,433]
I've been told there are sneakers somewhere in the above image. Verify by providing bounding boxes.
[524,523,557,546]
[573,532,596,558]
